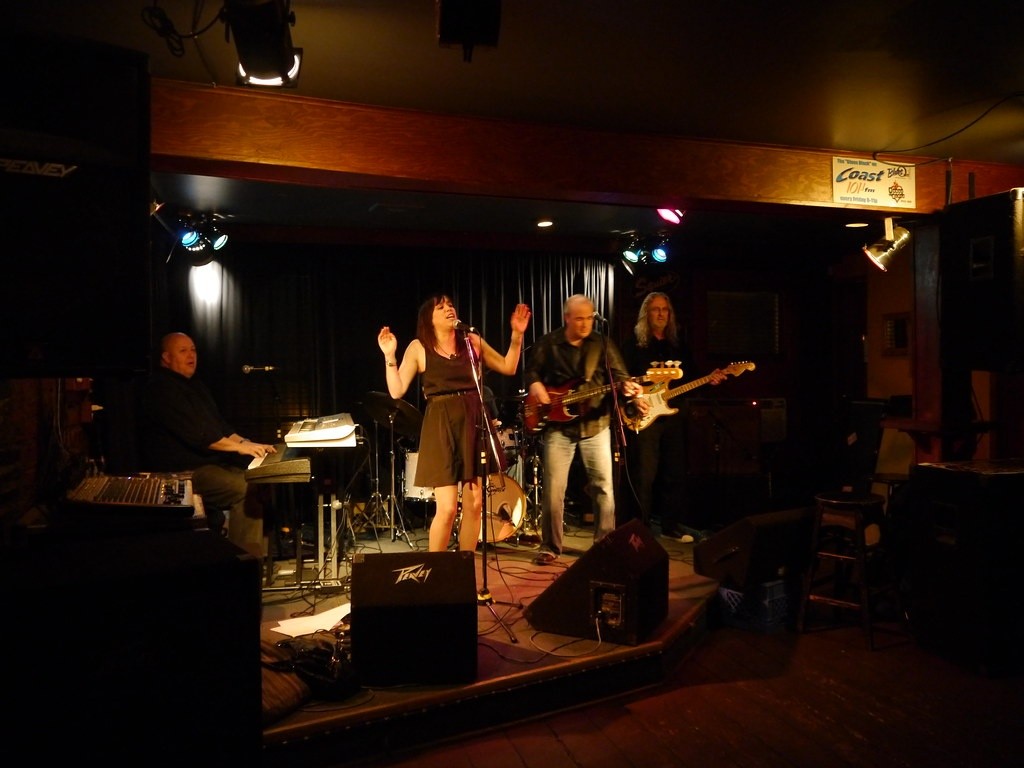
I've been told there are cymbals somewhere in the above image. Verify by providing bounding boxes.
[366,388,423,439]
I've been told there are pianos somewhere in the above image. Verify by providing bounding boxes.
[241,412,361,594]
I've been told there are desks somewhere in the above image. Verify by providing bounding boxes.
[796,493,897,634]
[907,459,1024,683]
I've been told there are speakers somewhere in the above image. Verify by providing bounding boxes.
[938,188,1024,373]
[693,507,820,579]
[350,550,478,684]
[523,520,670,646]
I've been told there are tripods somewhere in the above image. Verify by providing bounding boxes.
[343,413,579,643]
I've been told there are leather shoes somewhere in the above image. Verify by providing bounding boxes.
[535,553,558,564]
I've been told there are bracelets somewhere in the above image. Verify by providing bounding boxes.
[384,359,397,366]
[240,438,250,444]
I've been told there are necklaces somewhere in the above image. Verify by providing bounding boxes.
[437,341,458,360]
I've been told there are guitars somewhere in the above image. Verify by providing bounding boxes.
[621,360,756,432]
[523,358,685,434]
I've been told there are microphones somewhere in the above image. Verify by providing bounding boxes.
[452,320,477,333]
[243,365,276,373]
[591,312,608,323]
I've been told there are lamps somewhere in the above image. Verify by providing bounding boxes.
[622,237,648,262]
[196,217,228,249]
[622,259,645,275]
[651,232,672,263]
[175,219,199,248]
[863,217,911,272]
[220,0,302,89]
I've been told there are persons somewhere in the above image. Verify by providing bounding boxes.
[378,294,531,552]
[525,294,644,565]
[133,331,277,558]
[619,291,727,543]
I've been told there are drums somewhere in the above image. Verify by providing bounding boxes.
[498,426,522,453]
[399,448,461,503]
[455,472,528,544]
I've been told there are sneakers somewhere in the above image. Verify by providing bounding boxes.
[660,524,694,543]
